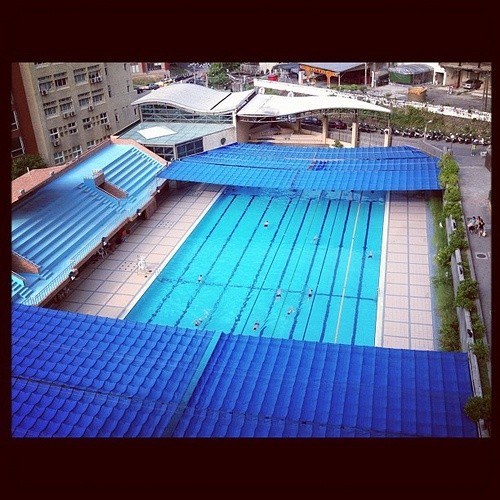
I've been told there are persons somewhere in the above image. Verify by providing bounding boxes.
[96,243,114,259]
[468,216,476,235]
[308,290,313,300]
[477,219,485,238]
[192,312,208,328]
[53,286,71,304]
[252,320,261,332]
[264,221,269,229]
[193,275,202,286]
[476,216,487,236]
[275,289,281,300]
[287,307,294,317]
[368,250,373,260]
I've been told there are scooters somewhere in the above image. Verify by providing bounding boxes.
[358,122,491,145]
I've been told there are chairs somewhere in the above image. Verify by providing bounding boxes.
[156,144,445,190]
[12,302,476,437]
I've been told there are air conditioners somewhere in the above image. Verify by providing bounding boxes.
[106,124,110,129]
[41,89,48,95]
[53,140,60,147]
[63,112,70,118]
[70,110,76,117]
[90,78,96,83]
[88,105,94,111]
[96,76,102,82]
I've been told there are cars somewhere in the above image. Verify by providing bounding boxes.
[300,116,321,126]
[463,79,481,89]
[329,119,346,129]
